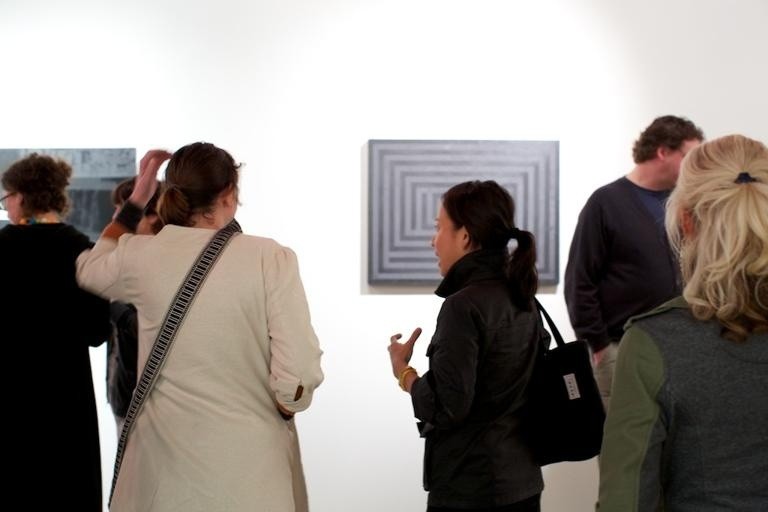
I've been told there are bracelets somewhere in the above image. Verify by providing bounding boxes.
[398,363,417,392]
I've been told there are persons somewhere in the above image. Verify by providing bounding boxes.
[75,142,324,512]
[563,113,708,420]
[387,179,551,512]
[104,174,166,447]
[0,154,111,510]
[593,134,768,512]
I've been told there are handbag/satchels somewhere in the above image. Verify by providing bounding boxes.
[534,296,606,466]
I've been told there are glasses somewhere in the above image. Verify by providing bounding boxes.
[0,191,20,209]
